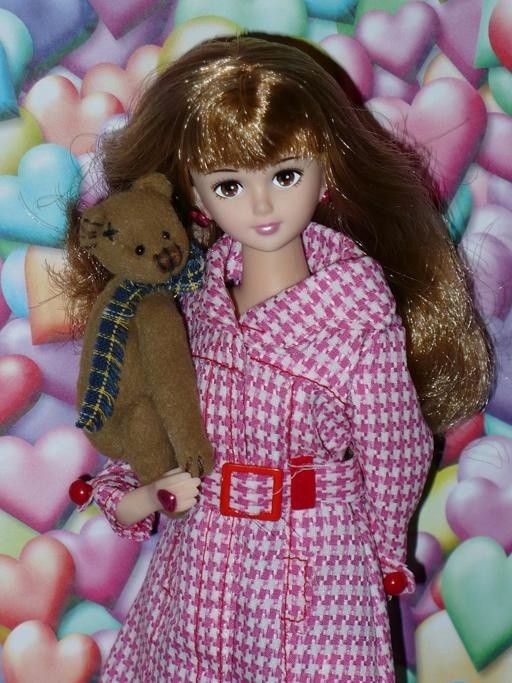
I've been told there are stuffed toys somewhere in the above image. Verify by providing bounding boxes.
[78,174,215,521]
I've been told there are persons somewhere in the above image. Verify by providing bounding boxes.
[44,35,499,683]
